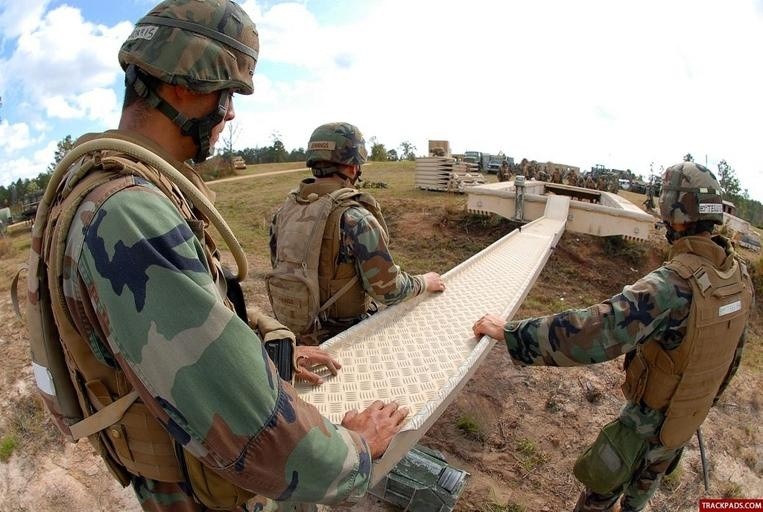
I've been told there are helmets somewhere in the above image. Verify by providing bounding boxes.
[118,0,259,95]
[305,122,368,168]
[659,163,724,225]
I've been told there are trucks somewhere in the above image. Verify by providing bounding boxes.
[228,154,247,170]
[485,152,515,174]
[462,149,484,169]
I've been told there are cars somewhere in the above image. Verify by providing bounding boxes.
[614,178,630,189]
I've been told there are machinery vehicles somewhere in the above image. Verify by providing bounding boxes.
[0,207,13,228]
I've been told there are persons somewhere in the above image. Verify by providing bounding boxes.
[14,2,412,512]
[498,159,619,196]
[471,161,756,510]
[264,121,447,345]
